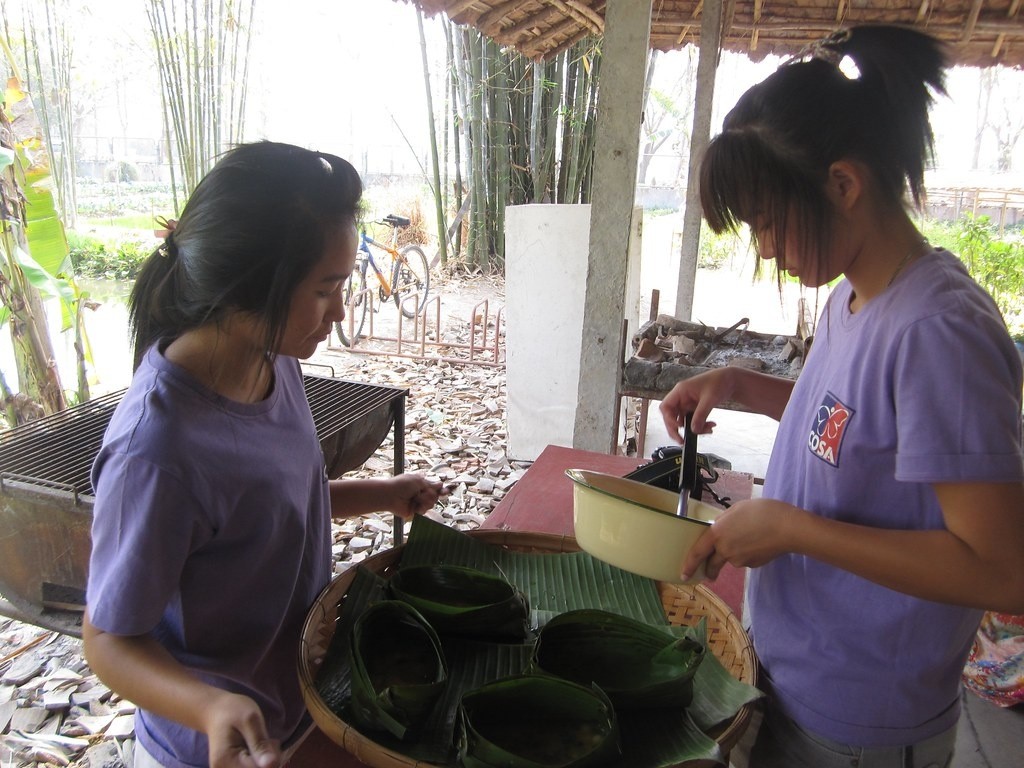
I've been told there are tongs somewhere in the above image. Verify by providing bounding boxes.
[712,317,750,350]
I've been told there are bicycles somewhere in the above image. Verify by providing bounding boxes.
[334,213,430,348]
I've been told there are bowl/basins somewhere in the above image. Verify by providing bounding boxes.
[564,468,726,587]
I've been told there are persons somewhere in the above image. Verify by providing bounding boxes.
[660,20,1024,768]
[83,140,448,768]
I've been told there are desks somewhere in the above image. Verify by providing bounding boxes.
[281,444,754,768]
